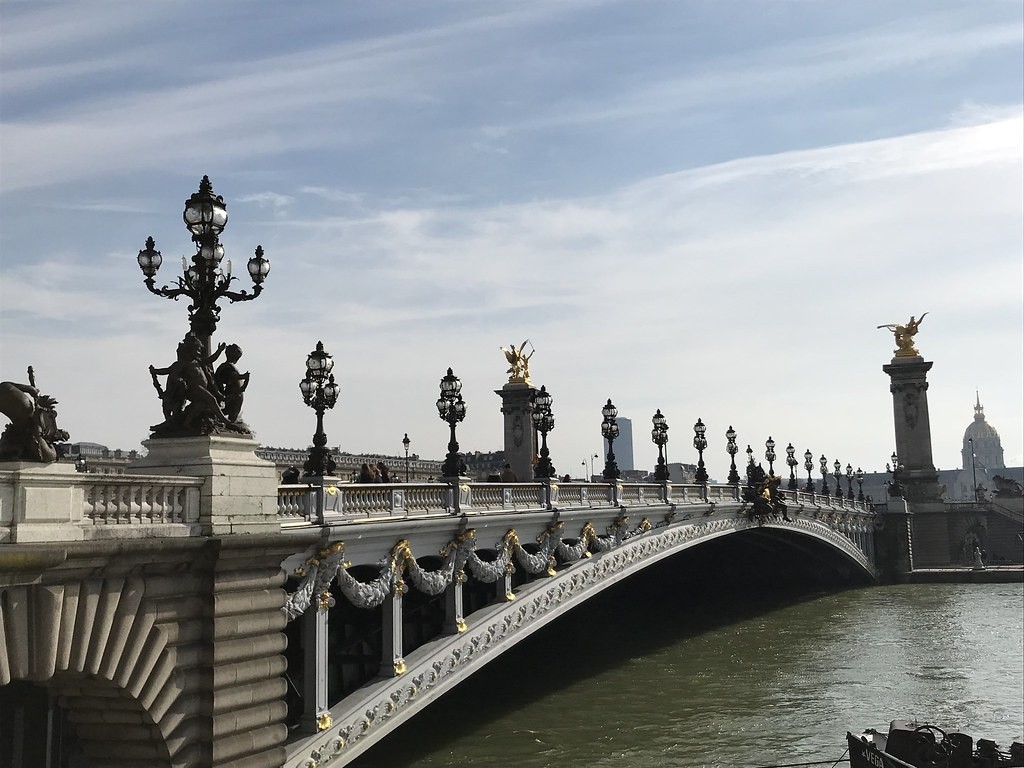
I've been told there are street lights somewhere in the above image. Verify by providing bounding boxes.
[968,437,979,501]
[726,425,740,485]
[582,459,588,482]
[886,452,906,495]
[300,340,341,481]
[845,463,855,499]
[745,444,753,467]
[834,459,843,495]
[693,418,710,485]
[651,408,670,482]
[857,466,865,499]
[819,454,830,495]
[591,452,598,483]
[602,398,622,481]
[531,384,557,482]
[138,173,269,442]
[403,434,412,481]
[75,453,86,471]
[765,436,777,480]
[436,368,470,482]
[786,441,800,489]
[804,450,816,493]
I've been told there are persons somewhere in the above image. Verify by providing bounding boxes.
[0,382,57,462]
[428,475,434,483]
[369,463,383,484]
[501,464,518,482]
[741,473,792,525]
[562,474,572,483]
[280,464,301,483]
[992,475,1023,497]
[391,473,400,482]
[359,463,376,483]
[377,462,389,483]
[156,337,250,431]
